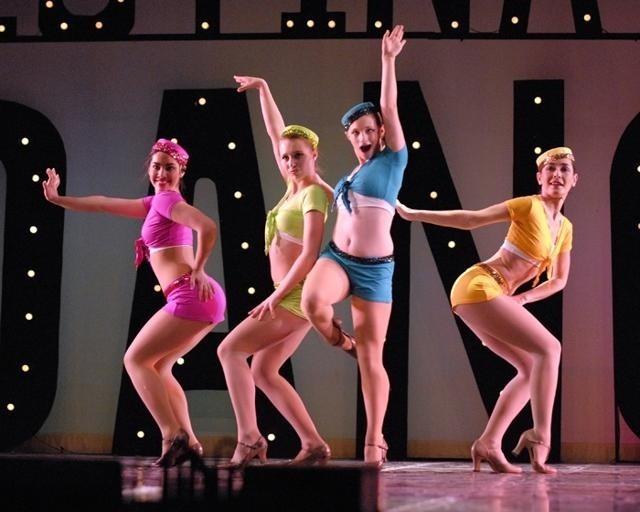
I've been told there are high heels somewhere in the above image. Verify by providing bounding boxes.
[213,434,269,469]
[152,428,202,465]
[330,318,360,361]
[286,442,330,466]
[362,433,389,469]
[511,427,558,474]
[470,435,523,475]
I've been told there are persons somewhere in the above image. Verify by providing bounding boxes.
[41,138,229,466]
[214,73,331,467]
[299,24,410,474]
[394,145,583,475]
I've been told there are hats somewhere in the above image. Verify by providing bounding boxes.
[150,138,190,170]
[340,101,375,132]
[535,147,576,171]
[280,124,319,150]
